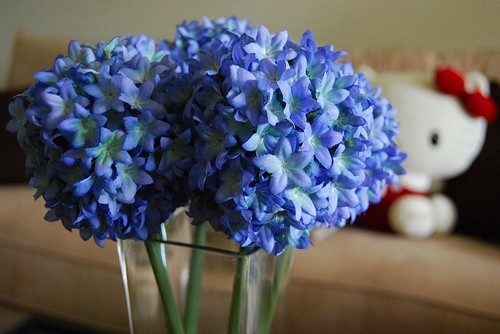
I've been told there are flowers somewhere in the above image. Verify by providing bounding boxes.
[7,16,408,334]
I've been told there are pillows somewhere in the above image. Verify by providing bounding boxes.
[444,80,499,247]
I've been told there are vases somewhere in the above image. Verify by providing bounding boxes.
[117,221,292,334]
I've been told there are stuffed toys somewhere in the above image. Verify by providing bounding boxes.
[354,62,495,238]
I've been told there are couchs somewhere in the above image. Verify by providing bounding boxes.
[0,25,500,334]
